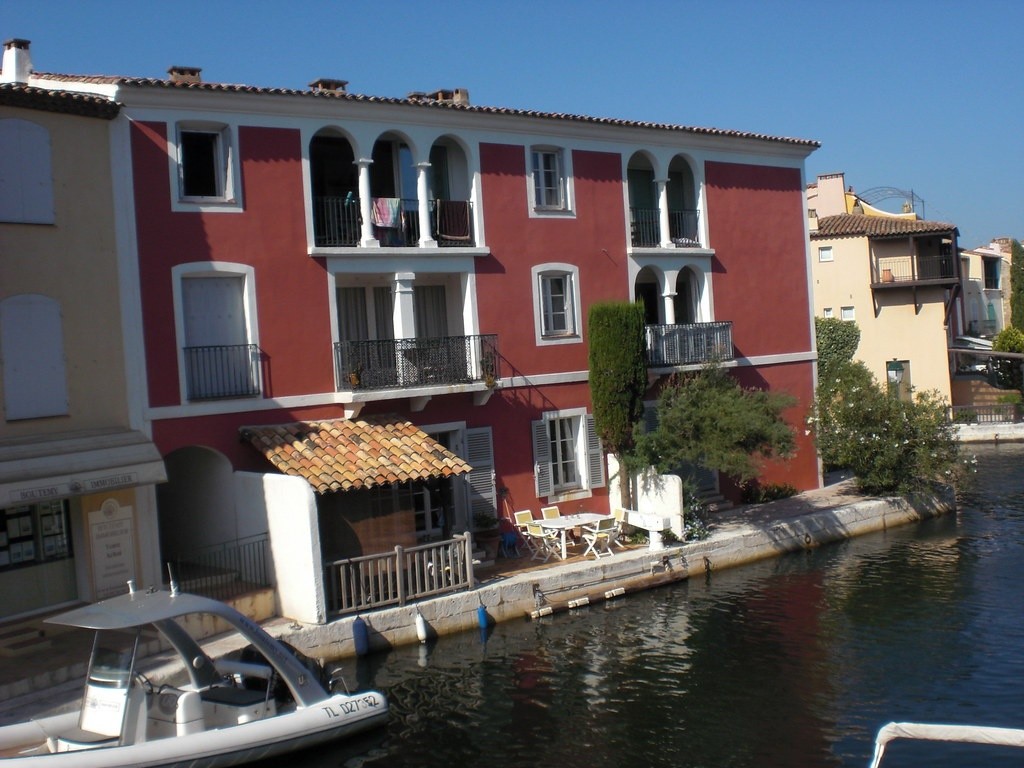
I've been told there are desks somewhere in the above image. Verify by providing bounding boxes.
[532,512,613,559]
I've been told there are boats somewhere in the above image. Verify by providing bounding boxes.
[0,559,395,768]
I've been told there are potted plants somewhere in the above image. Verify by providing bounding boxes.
[347,364,364,386]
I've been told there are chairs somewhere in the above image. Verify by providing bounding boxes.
[512,506,630,559]
